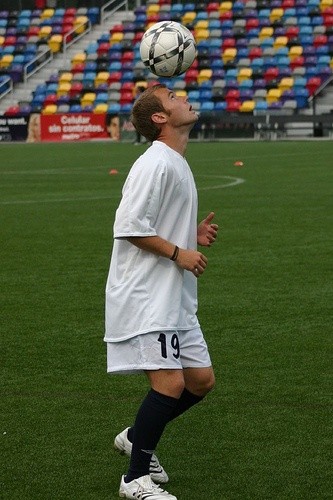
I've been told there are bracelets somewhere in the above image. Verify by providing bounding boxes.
[170,245,179,261]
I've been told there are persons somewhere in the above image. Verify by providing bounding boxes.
[133,85,147,143]
[26,113,40,142]
[105,114,120,140]
[103,83,219,500]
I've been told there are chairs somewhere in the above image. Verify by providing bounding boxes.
[2,0,333,115]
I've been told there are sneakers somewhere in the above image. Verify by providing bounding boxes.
[118,473,177,500]
[113,426,169,484]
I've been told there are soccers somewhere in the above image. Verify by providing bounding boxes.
[139,19,197,79]
